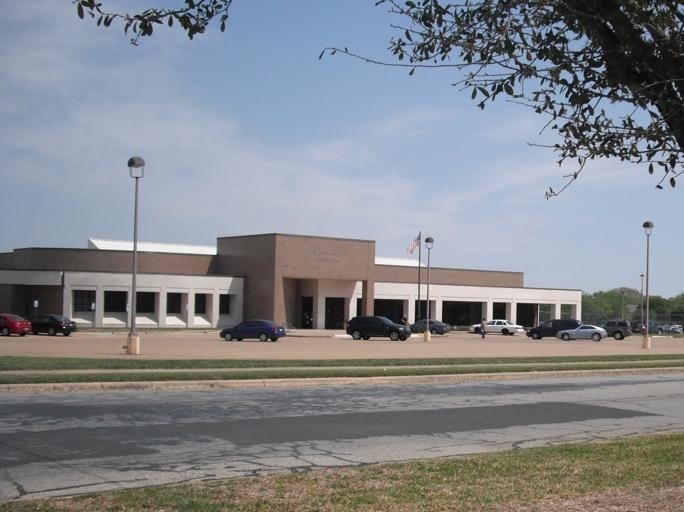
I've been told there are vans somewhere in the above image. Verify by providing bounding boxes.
[526,318,582,340]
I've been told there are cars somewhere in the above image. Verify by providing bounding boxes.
[469,319,525,335]
[0,314,31,336]
[631,321,684,336]
[219,319,286,343]
[408,319,451,335]
[557,323,608,342]
[28,314,78,336]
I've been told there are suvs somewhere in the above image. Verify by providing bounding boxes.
[599,320,633,340]
[345,316,411,342]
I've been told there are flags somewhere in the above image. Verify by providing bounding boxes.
[406,236,419,255]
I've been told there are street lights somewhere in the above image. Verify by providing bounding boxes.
[640,274,644,328]
[424,236,434,331]
[642,220,654,349]
[126,154,146,355]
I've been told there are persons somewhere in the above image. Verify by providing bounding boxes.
[478,317,486,339]
[400,312,408,325]
